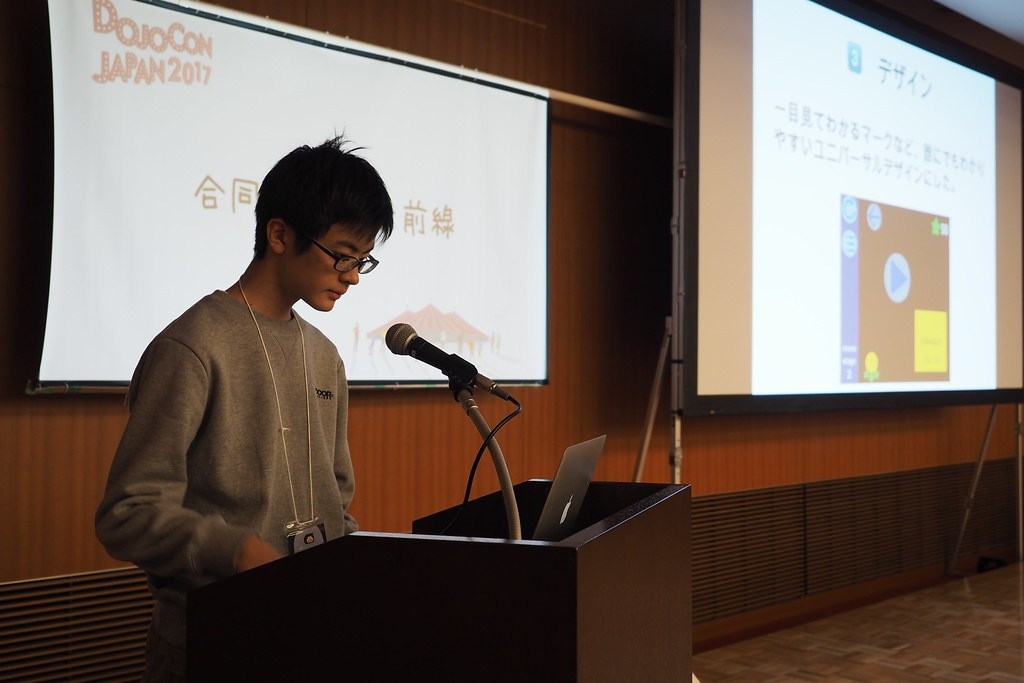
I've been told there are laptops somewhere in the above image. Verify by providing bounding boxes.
[533,434,607,541]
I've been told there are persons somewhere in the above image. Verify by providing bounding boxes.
[94,134,394,683]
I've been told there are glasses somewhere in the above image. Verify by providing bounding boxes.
[310,239,379,274]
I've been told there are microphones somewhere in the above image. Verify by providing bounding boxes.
[386,323,510,400]
